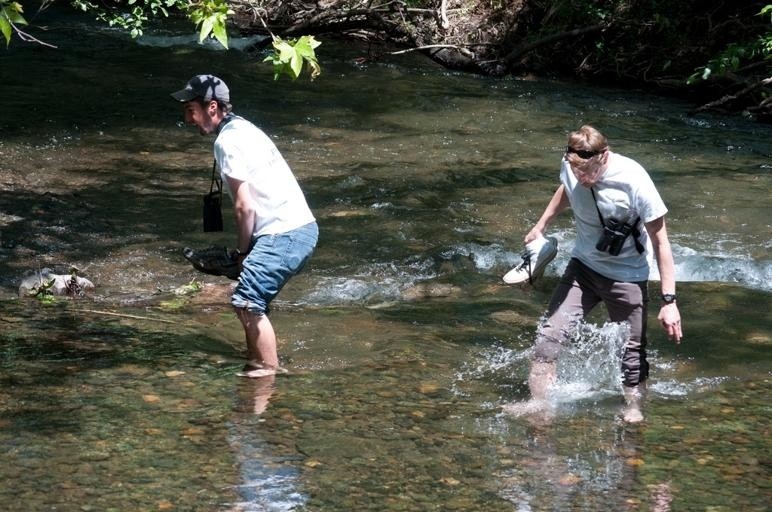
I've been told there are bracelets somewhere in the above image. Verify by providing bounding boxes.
[234,246,247,257]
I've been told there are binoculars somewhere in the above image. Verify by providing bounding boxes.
[595,217,632,256]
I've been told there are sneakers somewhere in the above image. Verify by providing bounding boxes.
[502,236,558,286]
[182,243,242,281]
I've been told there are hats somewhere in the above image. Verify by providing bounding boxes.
[170,74,231,105]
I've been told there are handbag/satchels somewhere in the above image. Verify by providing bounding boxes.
[202,186,226,234]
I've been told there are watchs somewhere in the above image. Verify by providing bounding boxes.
[661,293,678,304]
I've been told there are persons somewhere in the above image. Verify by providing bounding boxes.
[496,120,685,423]
[219,365,309,510]
[168,74,320,381]
[486,407,675,512]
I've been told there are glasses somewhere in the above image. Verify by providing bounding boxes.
[566,144,605,160]
